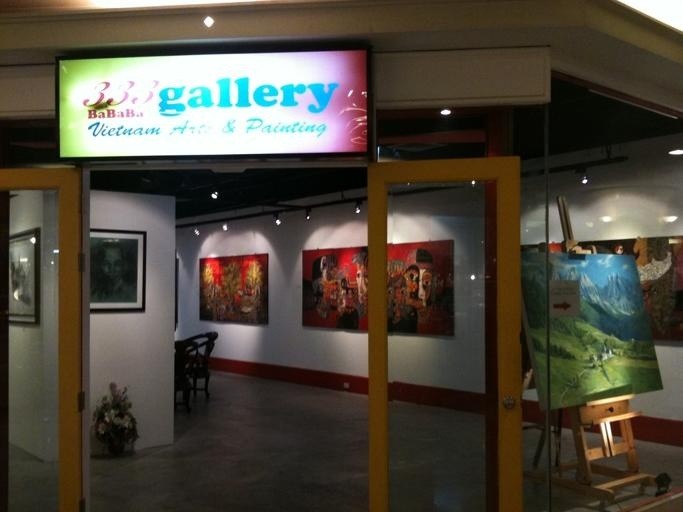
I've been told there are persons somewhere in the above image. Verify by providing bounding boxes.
[92,243,136,302]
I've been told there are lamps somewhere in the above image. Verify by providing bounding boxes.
[208,190,363,231]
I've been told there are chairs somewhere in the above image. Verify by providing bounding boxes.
[173,330,218,415]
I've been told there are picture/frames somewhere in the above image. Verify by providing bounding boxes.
[87,227,146,313]
[7,226,42,326]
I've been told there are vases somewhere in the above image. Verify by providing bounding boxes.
[108,436,124,456]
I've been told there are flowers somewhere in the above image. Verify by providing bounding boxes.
[91,381,139,446]
